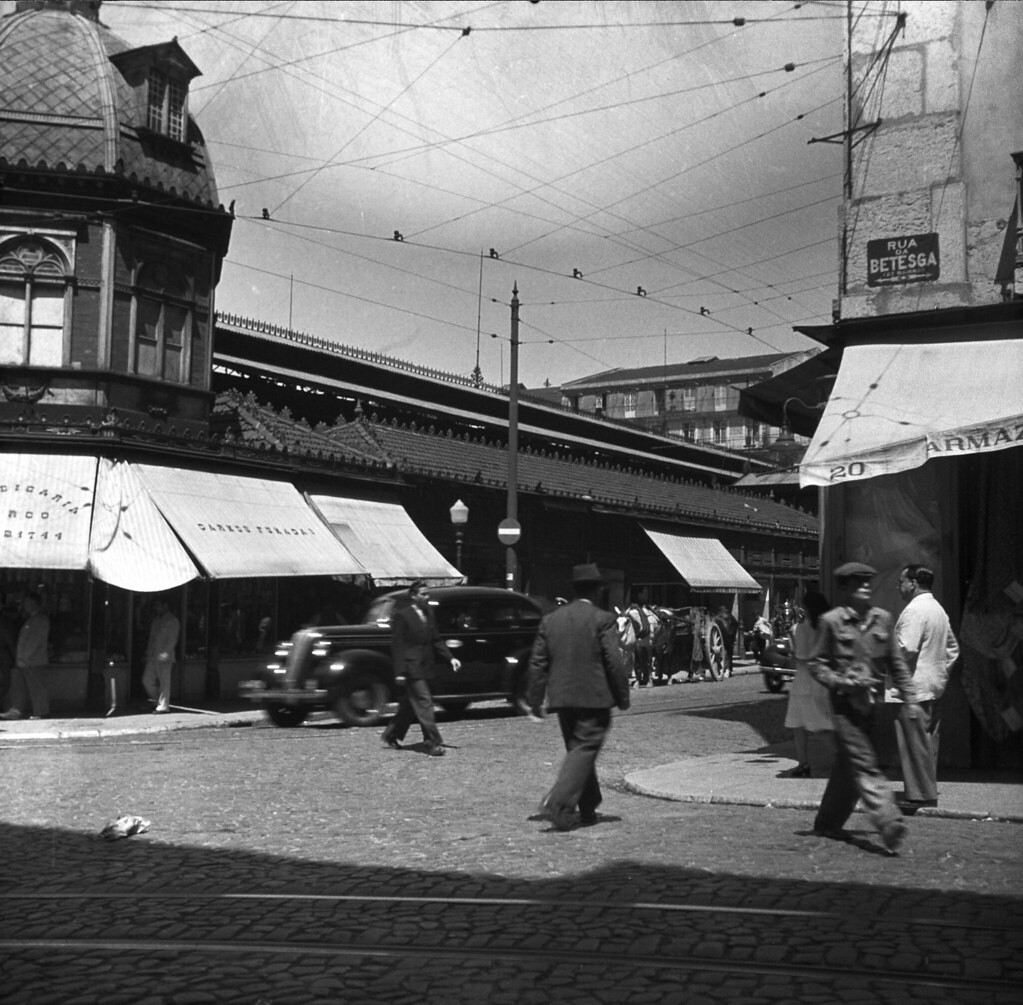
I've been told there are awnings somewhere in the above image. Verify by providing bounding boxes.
[799,341,1023,490]
[0,452,465,592]
[644,527,763,594]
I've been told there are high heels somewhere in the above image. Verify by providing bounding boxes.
[790,764,812,777]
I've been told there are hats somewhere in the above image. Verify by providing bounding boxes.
[832,563,877,578]
[562,562,611,589]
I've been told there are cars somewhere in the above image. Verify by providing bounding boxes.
[239,586,549,727]
[757,637,797,693]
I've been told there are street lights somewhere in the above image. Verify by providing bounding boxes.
[451,499,468,573]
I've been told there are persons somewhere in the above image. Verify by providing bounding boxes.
[142,595,180,714]
[526,578,631,830]
[290,596,374,634]
[814,562,916,855]
[919,535,1023,769]
[785,592,835,778]
[883,564,959,809]
[748,600,806,664]
[719,606,738,676]
[0,592,50,719]
[381,581,461,756]
[246,605,273,653]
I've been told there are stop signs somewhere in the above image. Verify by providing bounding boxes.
[498,519,522,545]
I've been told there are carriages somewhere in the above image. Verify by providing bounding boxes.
[615,604,726,688]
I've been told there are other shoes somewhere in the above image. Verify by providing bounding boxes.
[814,824,847,839]
[895,797,937,812]
[152,710,169,714]
[538,797,576,828]
[580,808,597,822]
[380,733,401,750]
[883,821,907,849]
[424,743,445,755]
[0,706,45,719]
